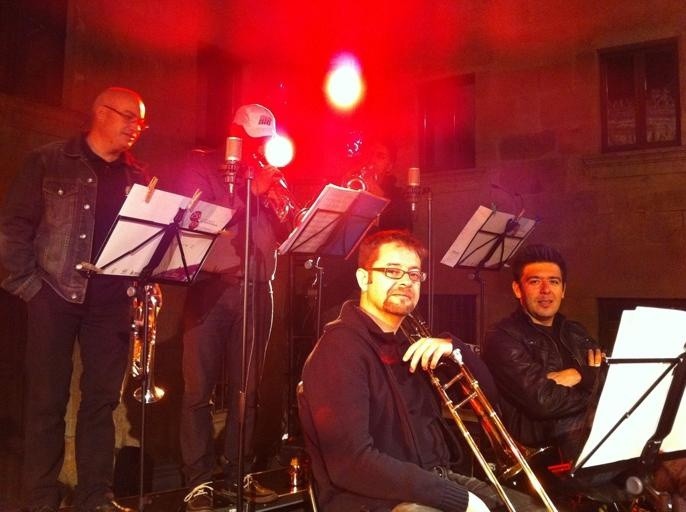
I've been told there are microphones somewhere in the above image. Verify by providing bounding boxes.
[225,137,240,194]
[409,168,419,211]
[252,152,289,190]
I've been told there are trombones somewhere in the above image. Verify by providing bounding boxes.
[400,311,560,511]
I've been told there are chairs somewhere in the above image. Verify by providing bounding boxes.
[307,477,320,512]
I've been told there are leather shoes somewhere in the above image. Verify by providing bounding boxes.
[70,494,137,512]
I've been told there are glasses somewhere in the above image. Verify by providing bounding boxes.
[355,266,427,283]
[104,103,148,131]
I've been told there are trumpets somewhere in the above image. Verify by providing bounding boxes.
[130,282,165,405]
[251,150,313,230]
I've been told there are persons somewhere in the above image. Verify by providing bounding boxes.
[173,103,279,510]
[299,229,552,512]
[478,242,617,500]
[0,87,151,511]
[313,136,412,346]
